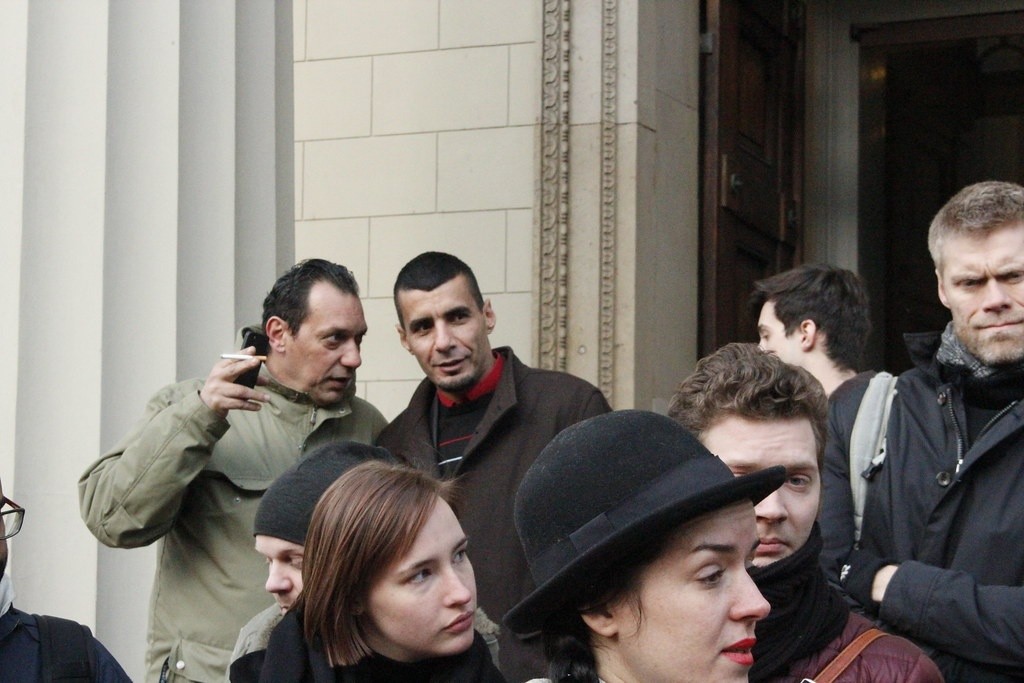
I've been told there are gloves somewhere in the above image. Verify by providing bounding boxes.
[840,548,900,615]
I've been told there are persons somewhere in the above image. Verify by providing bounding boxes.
[751,258,907,624]
[0,480,135,683]
[366,251,624,683]
[659,340,953,683]
[839,177,1024,683]
[503,408,787,683]
[222,440,508,683]
[228,456,514,683]
[77,258,390,683]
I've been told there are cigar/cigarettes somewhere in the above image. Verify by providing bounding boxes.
[220,354,268,362]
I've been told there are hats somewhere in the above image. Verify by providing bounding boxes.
[253,441,400,546]
[502,409,787,634]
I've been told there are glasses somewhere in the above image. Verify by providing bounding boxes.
[0,497,25,540]
